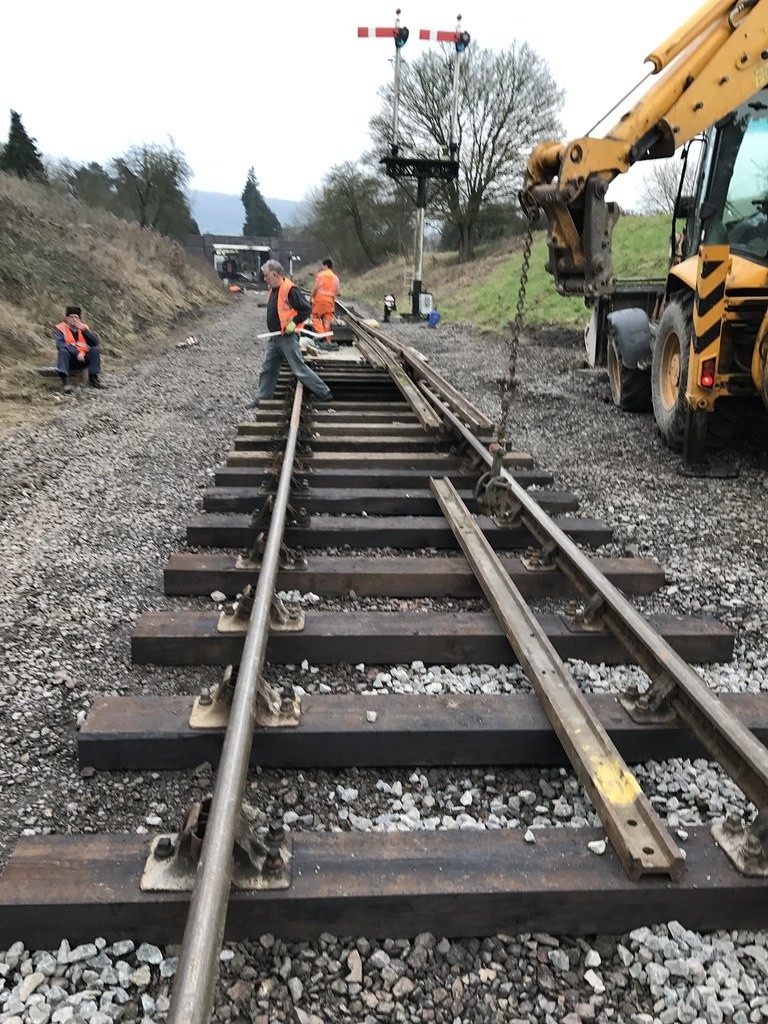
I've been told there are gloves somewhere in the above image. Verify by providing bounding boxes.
[285,321,296,336]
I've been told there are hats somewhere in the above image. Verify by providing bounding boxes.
[65,306,81,314]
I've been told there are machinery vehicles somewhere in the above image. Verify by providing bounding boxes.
[516,0,768,455]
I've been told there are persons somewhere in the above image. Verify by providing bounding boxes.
[54,306,108,393]
[244,260,334,409]
[310,259,341,344]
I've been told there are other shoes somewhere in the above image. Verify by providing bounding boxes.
[89,374,107,389]
[304,391,333,402]
[62,381,71,394]
[243,399,258,408]
